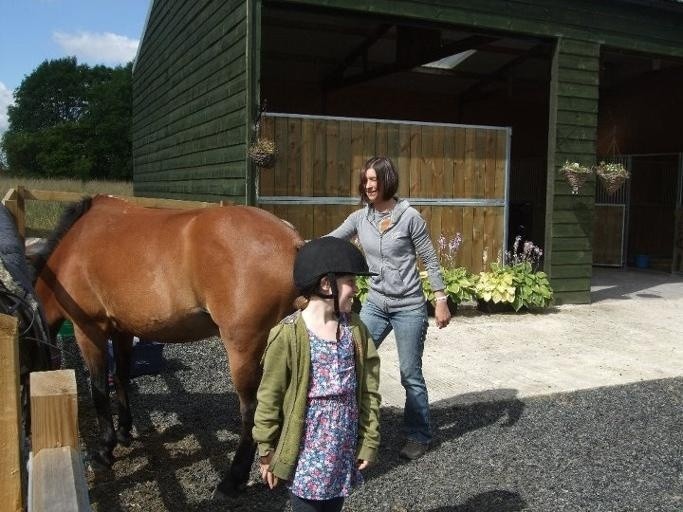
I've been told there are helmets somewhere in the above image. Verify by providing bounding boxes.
[293,237,378,290]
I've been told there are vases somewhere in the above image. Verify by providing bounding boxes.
[428,296,457,317]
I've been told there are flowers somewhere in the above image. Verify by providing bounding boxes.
[415,231,553,313]
[564,159,628,174]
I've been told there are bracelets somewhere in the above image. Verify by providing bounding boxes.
[434,296,448,303]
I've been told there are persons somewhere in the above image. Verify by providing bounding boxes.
[251,234,383,512]
[305,156,453,461]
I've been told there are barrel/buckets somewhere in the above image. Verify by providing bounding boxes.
[636,255,649,268]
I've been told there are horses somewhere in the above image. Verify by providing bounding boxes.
[26,193,309,503]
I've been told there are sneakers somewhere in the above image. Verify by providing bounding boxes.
[399,440,428,459]
